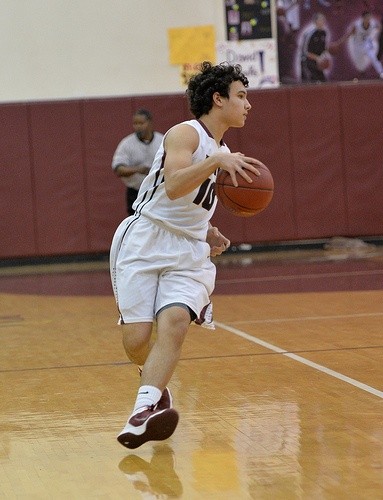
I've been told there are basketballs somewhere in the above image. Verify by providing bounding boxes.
[214,156,276,219]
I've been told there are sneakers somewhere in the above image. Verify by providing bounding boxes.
[116,405,179,449]
[138,365,172,411]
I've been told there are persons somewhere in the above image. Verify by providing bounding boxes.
[330,10,382,79]
[293,11,334,83]
[109,60,269,449]
[111,109,164,216]
